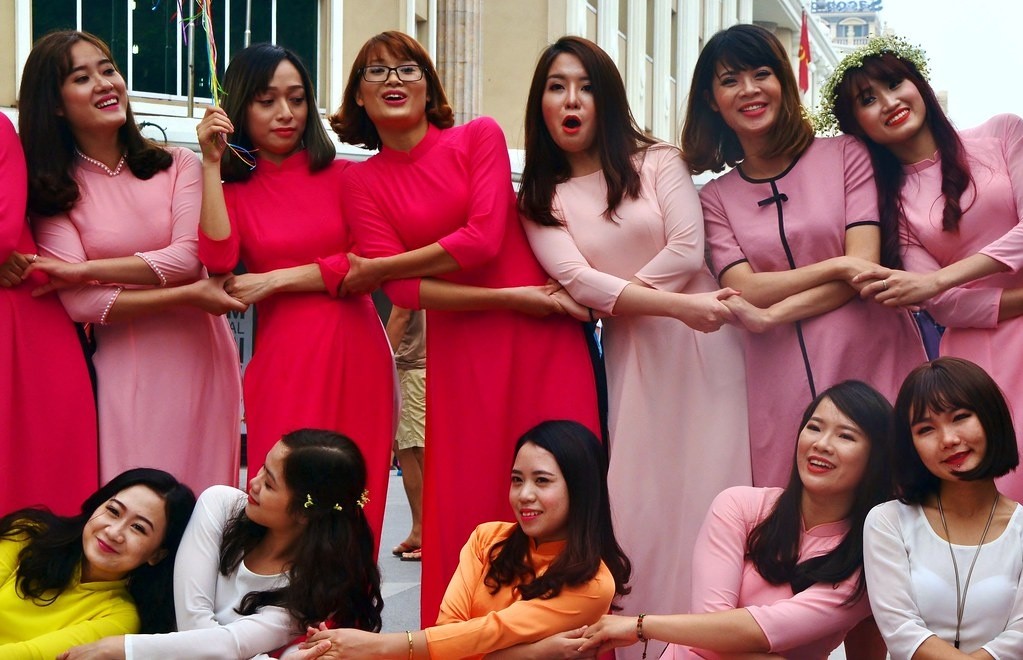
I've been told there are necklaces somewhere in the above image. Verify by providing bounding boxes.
[936,492,1000,649]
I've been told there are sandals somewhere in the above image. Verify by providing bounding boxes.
[392,541,422,555]
[400,548,423,561]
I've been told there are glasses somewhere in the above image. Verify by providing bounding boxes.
[358,64,424,83]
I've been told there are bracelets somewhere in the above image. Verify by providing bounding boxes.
[406,631,413,660]
[637,614,648,659]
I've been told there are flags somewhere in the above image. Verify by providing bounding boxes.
[798,12,811,93]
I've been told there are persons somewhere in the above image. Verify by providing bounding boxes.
[385,305,426,560]
[18,31,250,499]
[0,468,197,660]
[0,111,99,516]
[299,420,631,660]
[864,356,1023,660]
[820,36,1023,506]
[328,30,601,629]
[64,429,384,660]
[680,24,928,660]
[197,43,402,563]
[583,378,893,660]
[515,36,752,660]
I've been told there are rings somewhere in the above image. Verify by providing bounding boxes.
[33,254,37,261]
